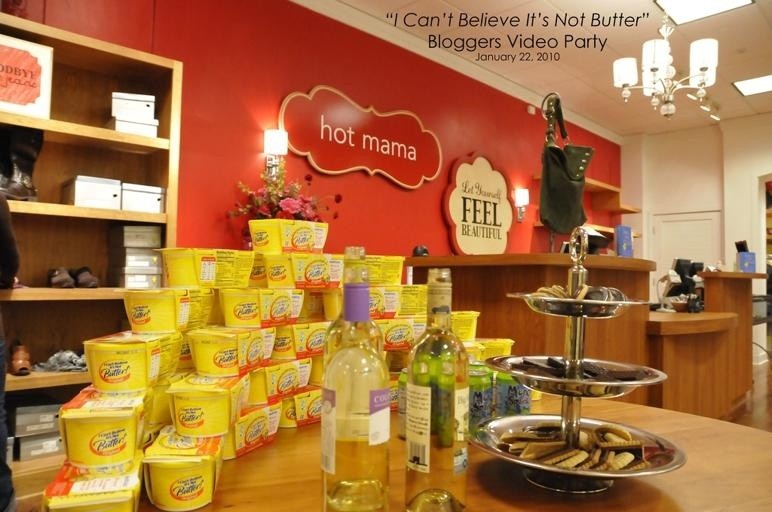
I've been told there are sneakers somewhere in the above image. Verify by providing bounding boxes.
[48,269,75,288]
[73,267,101,287]
[10,339,32,376]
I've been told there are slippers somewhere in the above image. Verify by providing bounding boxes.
[34,345,89,372]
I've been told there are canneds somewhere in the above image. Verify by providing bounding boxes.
[395,359,531,447]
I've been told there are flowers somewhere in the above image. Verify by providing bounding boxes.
[224,175,343,223]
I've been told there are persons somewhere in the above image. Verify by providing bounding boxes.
[0,195,21,512]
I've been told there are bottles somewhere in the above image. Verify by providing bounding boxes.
[320,243,388,368]
[318,280,392,511]
[403,265,472,509]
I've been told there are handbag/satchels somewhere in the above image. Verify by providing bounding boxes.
[537,144,594,235]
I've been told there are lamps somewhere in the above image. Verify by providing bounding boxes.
[609,1,725,122]
[515,188,529,224]
[261,128,288,182]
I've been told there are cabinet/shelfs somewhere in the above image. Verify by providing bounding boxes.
[0,12,185,512]
[531,173,641,238]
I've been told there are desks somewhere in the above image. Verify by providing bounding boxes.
[138,381,771,510]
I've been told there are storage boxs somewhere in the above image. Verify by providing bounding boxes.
[104,91,159,137]
[104,224,164,289]
[60,175,166,215]
[2,396,64,464]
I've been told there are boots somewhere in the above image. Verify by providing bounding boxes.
[0,123,45,203]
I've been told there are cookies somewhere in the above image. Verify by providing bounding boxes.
[505,354,656,398]
[498,421,675,475]
[530,283,627,317]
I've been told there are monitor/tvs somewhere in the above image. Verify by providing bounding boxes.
[559,242,579,254]
[687,262,704,295]
[662,258,691,297]
